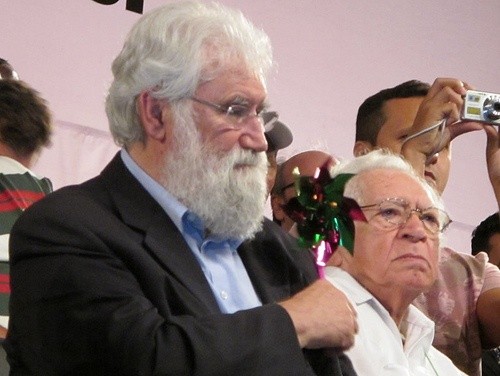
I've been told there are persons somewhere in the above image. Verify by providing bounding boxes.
[4,2,359,375]
[258,76,500,376]
[0,79,58,375]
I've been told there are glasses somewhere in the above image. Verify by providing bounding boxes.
[189,97,278,132]
[356,199,453,233]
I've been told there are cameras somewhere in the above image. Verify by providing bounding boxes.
[460,89,500,125]
